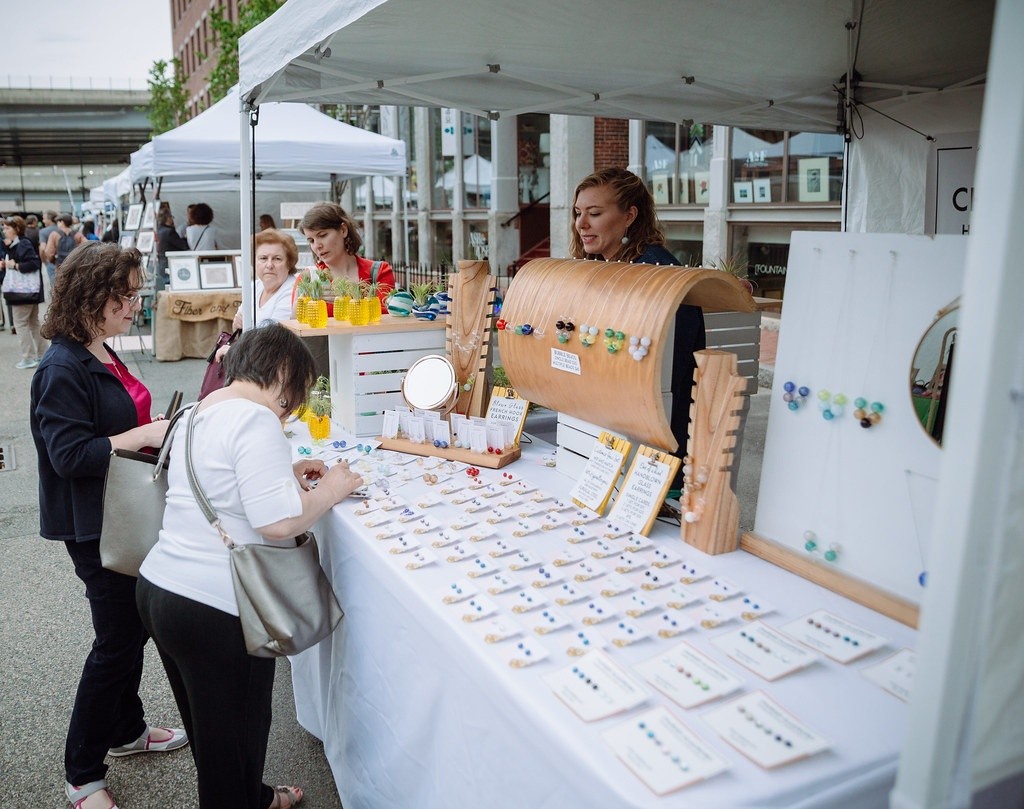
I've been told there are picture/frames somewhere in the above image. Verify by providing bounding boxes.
[135,230,155,253]
[732,181,753,204]
[199,262,235,289]
[233,254,243,288]
[119,235,134,251]
[124,203,144,230]
[752,177,772,204]
[671,171,689,204]
[651,171,669,204]
[693,170,709,204]
[144,201,161,229]
[168,256,201,291]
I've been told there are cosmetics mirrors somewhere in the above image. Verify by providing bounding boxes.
[401,355,460,416]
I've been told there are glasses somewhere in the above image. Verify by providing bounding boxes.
[110,293,142,307]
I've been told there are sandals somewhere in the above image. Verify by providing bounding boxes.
[268,785,304,809]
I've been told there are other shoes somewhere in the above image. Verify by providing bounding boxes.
[64,776,118,809]
[108,726,190,757]
[16,360,42,372]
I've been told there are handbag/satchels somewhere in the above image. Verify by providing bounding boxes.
[1,253,41,293]
[230,531,345,658]
[100,399,203,578]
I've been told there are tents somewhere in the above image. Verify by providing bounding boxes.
[81,86,410,292]
[644,129,846,181]
[238,0,1023,809]
[436,156,491,207]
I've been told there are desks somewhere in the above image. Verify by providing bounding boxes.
[286,429,920,809]
[153,289,243,358]
[283,314,446,436]
[112,292,153,363]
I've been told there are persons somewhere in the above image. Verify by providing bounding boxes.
[135,322,363,809]
[260,214,276,230]
[158,208,188,284]
[176,204,196,238]
[0,210,124,369]
[291,202,396,319]
[30,241,189,809]
[215,229,298,363]
[569,168,706,501]
[187,203,217,251]
